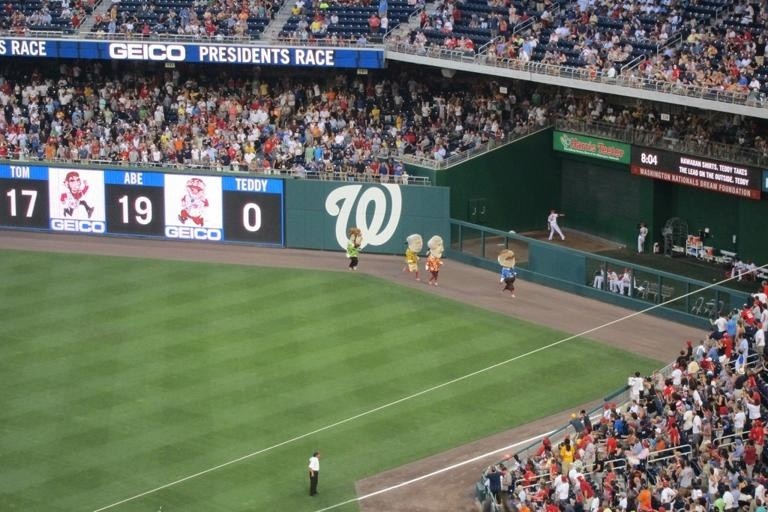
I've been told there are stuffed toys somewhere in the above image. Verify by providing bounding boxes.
[346,228,363,271]
[402,233,445,286]
[498,249,517,298]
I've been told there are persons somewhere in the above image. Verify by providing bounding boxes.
[593,264,636,294]
[637,220,648,254]
[0,0,768,184]
[731,256,757,282]
[485,281,768,512]
[309,451,321,496]
[547,208,566,241]
